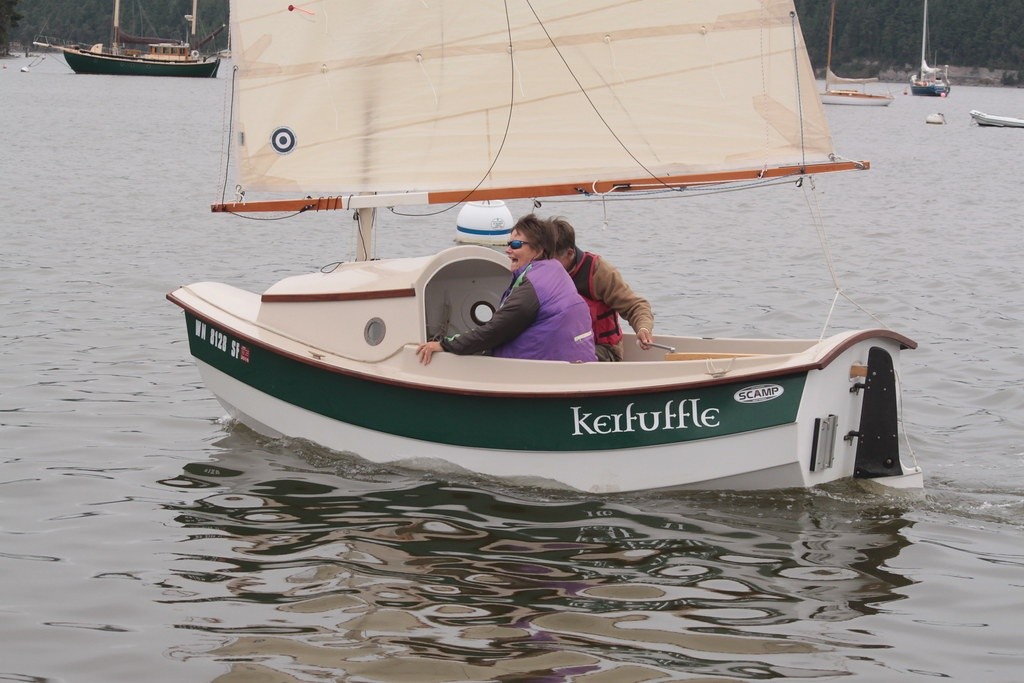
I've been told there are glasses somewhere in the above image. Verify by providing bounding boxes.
[506,240,530,248]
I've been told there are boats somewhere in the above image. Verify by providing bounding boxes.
[971,111,1024,128]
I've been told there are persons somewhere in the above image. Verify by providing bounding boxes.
[415,213,599,367]
[544,218,655,361]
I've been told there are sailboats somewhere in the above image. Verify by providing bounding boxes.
[819,0,896,107]
[910,0,951,97]
[173,0,926,503]
[32,0,226,79]
